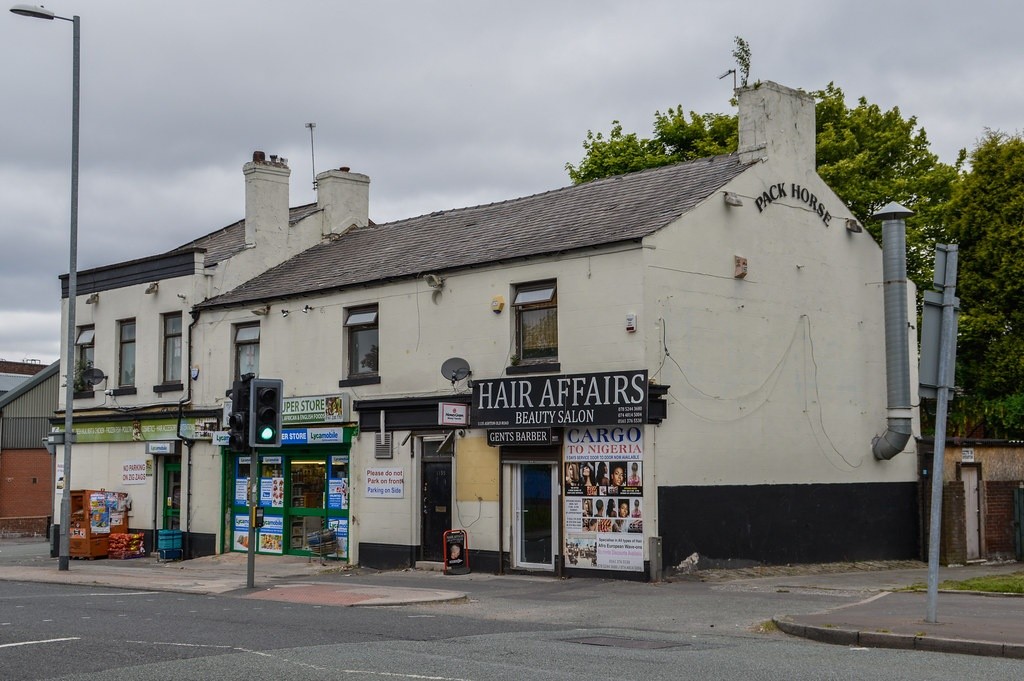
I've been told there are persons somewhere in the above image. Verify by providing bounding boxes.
[565,463,579,486]
[618,499,630,518]
[582,519,643,534]
[610,466,626,486]
[594,499,603,517]
[582,500,593,517]
[582,462,595,486]
[606,499,617,517]
[631,499,642,518]
[627,462,641,486]
[595,462,609,485]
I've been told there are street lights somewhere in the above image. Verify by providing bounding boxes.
[9,3,81,575]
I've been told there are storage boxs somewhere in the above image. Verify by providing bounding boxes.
[69,489,129,560]
[158,530,182,558]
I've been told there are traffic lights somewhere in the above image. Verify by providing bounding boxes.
[226,411,251,452]
[253,385,280,444]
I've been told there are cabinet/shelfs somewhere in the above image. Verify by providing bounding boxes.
[292,472,324,549]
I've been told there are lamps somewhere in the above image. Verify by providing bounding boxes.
[86,293,98,303]
[423,274,445,286]
[280,309,289,318]
[847,219,862,232]
[252,304,271,314]
[301,305,313,313]
[145,282,158,293]
[725,191,743,205]
